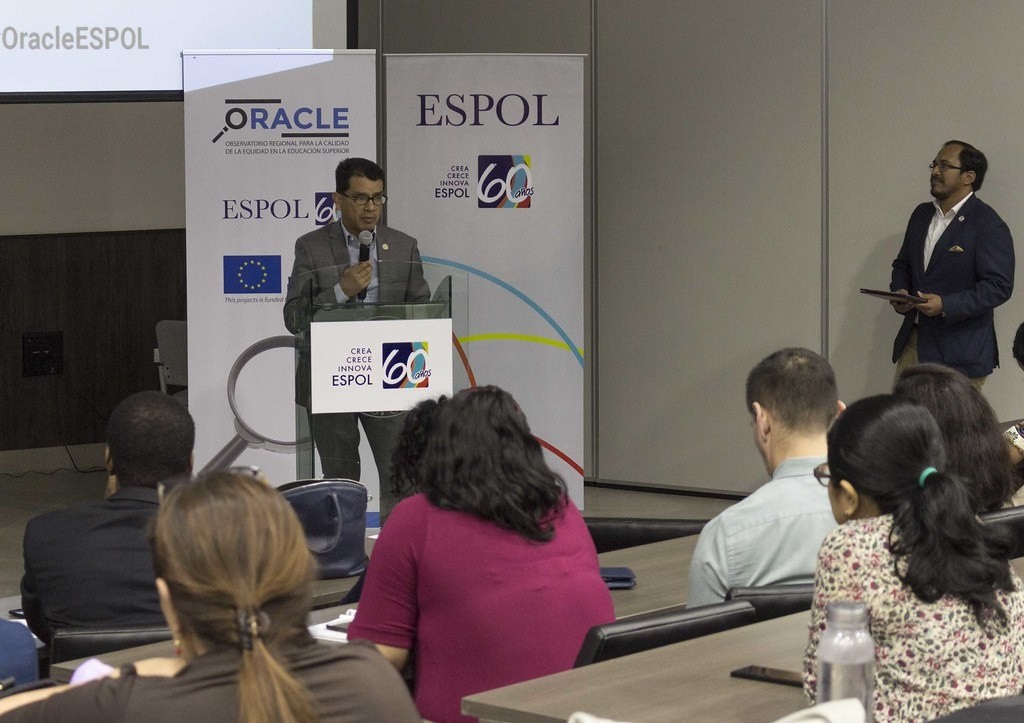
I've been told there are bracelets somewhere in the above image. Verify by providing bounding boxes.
[942,306,946,320]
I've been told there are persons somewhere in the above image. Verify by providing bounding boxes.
[889,140,1015,398]
[347,387,616,722]
[687,346,846,611]
[801,395,1024,723]
[21,392,196,646]
[892,363,1013,515]
[0,473,422,723]
[1001,323,1024,472]
[283,157,431,533]
[338,396,452,609]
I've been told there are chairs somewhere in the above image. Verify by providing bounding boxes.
[0,462,1024,723]
[53,507,1024,663]
[153,319,188,412]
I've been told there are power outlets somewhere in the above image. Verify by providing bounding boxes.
[23,331,64,380]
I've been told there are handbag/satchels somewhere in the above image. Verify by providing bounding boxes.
[274,478,370,579]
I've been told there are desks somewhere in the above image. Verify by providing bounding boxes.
[462,553,1024,721]
[49,526,785,683]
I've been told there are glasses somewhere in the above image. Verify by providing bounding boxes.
[814,462,838,488]
[340,193,388,206]
[928,162,963,171]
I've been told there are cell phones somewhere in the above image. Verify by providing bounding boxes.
[327,620,352,632]
[730,665,805,687]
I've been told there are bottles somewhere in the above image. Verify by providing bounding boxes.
[817,603,875,722]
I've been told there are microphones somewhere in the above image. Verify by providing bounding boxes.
[357,230,373,300]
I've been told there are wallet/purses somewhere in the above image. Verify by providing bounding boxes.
[598,567,636,589]
